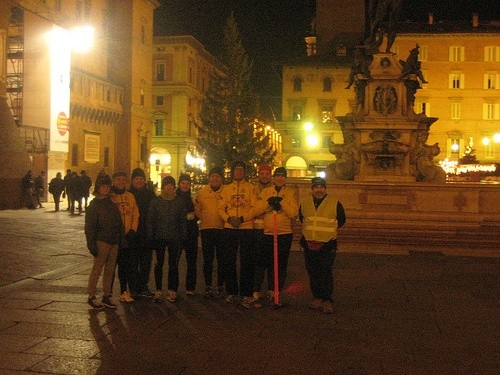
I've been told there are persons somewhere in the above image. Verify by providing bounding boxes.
[32,170,46,208]
[245,164,275,302]
[110,170,141,304]
[297,177,347,315]
[79,170,93,211]
[170,173,201,299]
[19,170,38,209]
[126,168,156,302]
[48,172,65,212]
[256,166,300,307]
[84,171,128,310]
[67,172,83,213]
[193,167,230,298]
[64,169,72,211]
[145,175,188,301]
[219,162,263,309]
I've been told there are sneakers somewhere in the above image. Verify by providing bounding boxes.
[120,292,134,302]
[138,289,154,297]
[167,292,178,302]
[321,300,334,313]
[226,295,239,303]
[87,297,104,309]
[215,284,223,295]
[102,295,117,308]
[203,288,213,293]
[310,298,323,308]
[242,298,253,307]
[253,292,261,306]
[154,292,164,303]
[269,291,282,305]
[186,289,195,295]
[107,292,116,302]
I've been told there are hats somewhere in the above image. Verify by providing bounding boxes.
[178,173,192,183]
[274,167,287,177]
[311,176,327,189]
[208,167,224,179]
[112,170,128,180]
[259,164,271,172]
[131,168,147,182]
[92,174,112,195]
[231,160,246,169]
